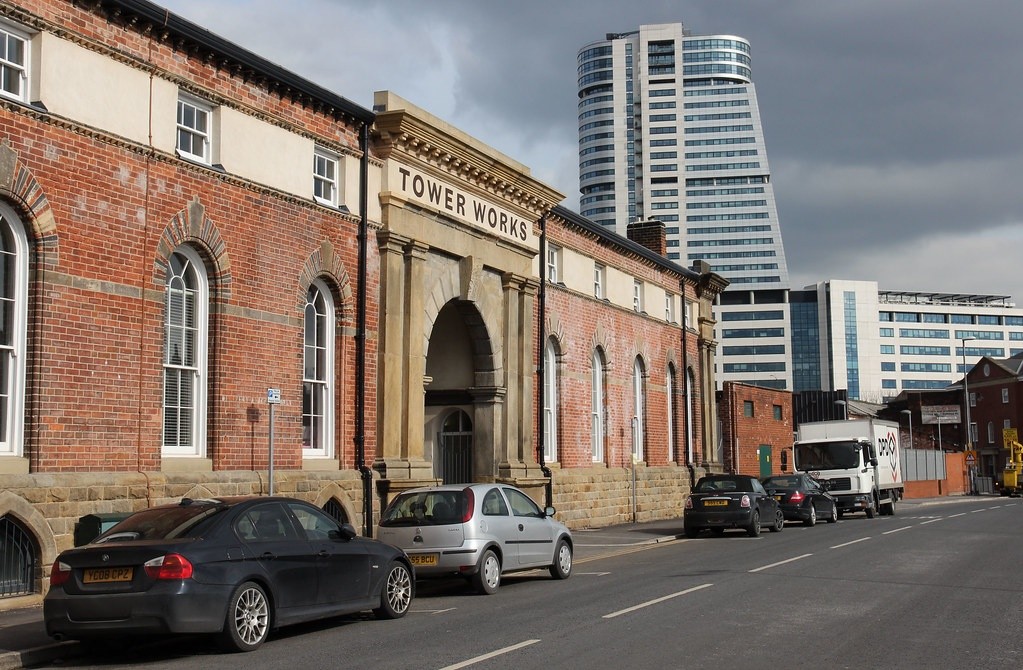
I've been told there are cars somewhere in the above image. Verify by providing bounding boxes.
[762,474,838,527]
[42,496,415,653]
[683,475,784,537]
[375,482,574,596]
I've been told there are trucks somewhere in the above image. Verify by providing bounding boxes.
[780,418,904,519]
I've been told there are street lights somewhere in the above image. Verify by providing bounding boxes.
[934,412,942,451]
[902,410,913,450]
[835,400,847,420]
[962,336,976,448]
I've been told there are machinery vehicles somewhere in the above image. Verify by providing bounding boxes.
[999,441,1023,496]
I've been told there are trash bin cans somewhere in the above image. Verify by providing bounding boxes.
[74,513,137,551]
